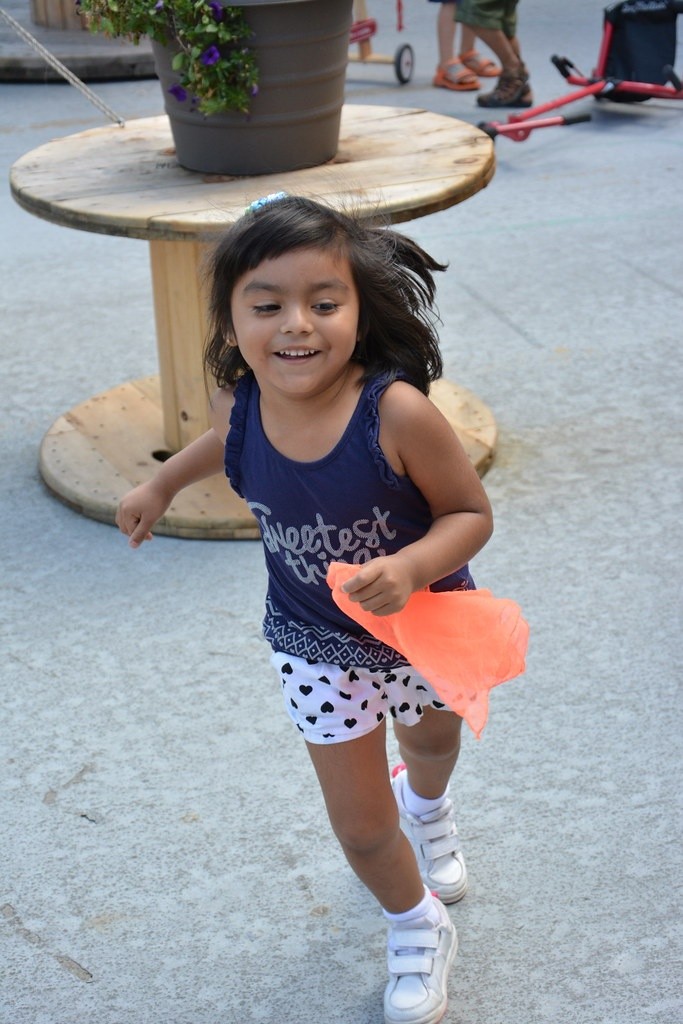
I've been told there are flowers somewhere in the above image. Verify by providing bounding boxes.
[75,0,259,121]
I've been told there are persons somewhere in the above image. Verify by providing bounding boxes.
[113,193,497,1023]
[430,0,532,106]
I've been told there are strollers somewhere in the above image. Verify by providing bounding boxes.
[477,0,682,143]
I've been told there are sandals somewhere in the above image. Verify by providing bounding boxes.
[458,50,502,77]
[434,59,480,90]
[478,83,532,107]
[491,60,530,104]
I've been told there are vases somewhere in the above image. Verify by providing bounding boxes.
[150,0,355,175]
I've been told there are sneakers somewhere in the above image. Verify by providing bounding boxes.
[390,769,468,905]
[383,893,459,1024]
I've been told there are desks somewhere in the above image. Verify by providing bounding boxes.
[6,101,499,543]
